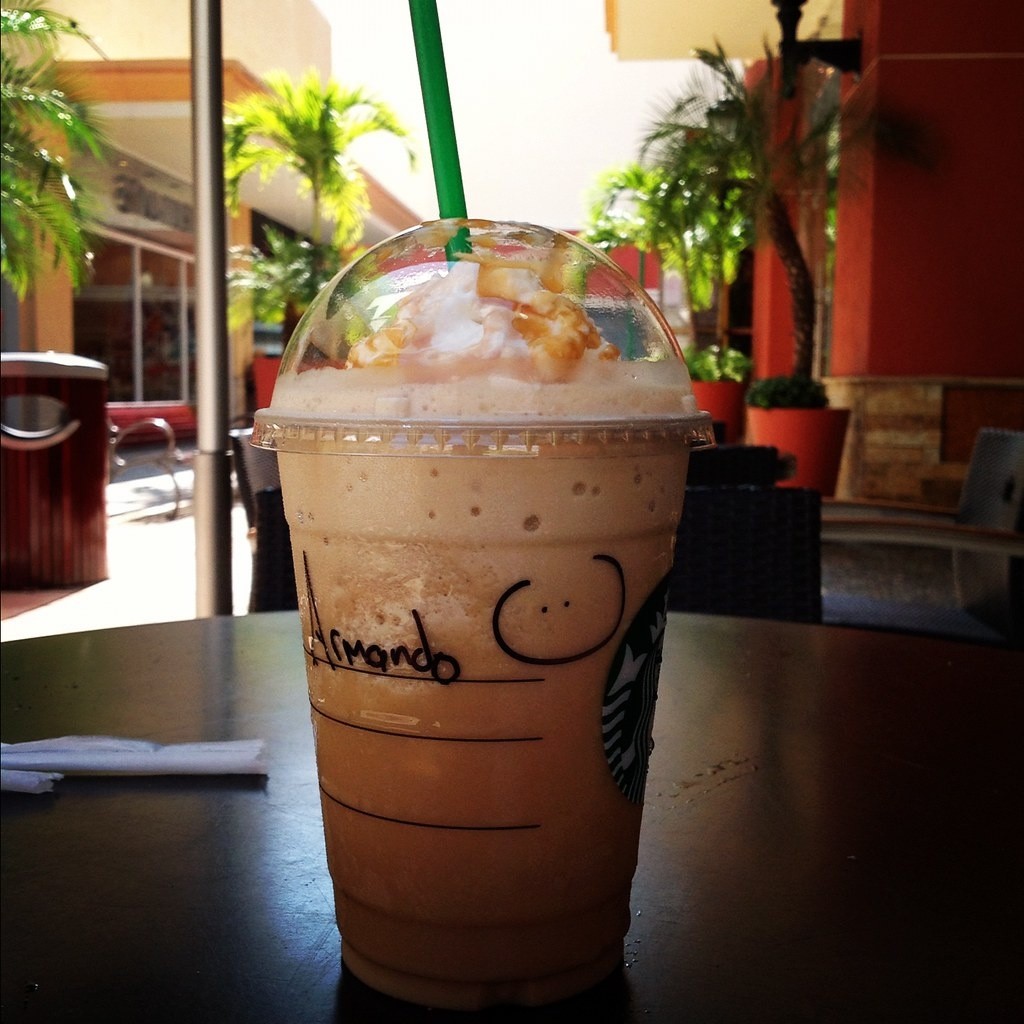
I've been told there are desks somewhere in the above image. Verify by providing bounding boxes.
[0,608,1024,1024]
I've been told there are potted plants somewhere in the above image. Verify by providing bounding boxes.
[566,36,941,500]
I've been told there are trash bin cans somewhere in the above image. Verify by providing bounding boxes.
[0,352,112,588]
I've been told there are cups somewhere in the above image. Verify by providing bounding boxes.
[249,220,716,1008]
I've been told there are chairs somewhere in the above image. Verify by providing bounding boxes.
[254,447,823,625]
